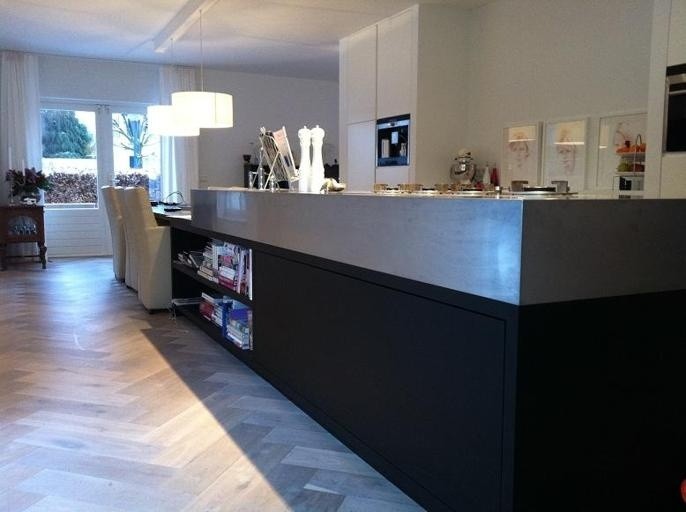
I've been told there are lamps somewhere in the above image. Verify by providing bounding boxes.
[144,33,199,138]
[169,5,235,132]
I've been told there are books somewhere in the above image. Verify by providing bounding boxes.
[173,237,252,352]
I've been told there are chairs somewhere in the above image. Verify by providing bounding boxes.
[112,184,138,292]
[123,185,172,316]
[0,203,48,273]
[99,187,124,281]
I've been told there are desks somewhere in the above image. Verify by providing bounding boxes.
[148,200,190,222]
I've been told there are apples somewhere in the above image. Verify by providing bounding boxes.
[617,163,644,172]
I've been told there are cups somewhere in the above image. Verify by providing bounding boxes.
[381,139,390,159]
[243,155,251,161]
[286,169,299,193]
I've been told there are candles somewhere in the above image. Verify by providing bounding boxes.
[8,146,12,171]
[21,159,25,177]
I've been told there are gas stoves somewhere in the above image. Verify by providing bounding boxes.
[373,179,578,196]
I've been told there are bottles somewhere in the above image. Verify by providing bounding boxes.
[400,144,406,157]
[297,125,311,194]
[449,152,498,185]
[310,124,326,195]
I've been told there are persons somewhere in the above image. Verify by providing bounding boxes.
[552,130,582,180]
[604,120,637,152]
[510,130,532,167]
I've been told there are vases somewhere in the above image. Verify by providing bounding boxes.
[20,194,39,202]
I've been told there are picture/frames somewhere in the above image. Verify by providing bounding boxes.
[498,119,542,187]
[591,107,644,193]
[543,112,591,193]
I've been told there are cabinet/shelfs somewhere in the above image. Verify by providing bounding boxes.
[377,0,457,190]
[641,1,685,198]
[338,28,378,191]
[168,222,253,366]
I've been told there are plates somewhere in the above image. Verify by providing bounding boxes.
[617,153,646,159]
[616,172,645,178]
[619,190,645,195]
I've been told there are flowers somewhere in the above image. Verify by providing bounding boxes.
[3,168,56,196]
